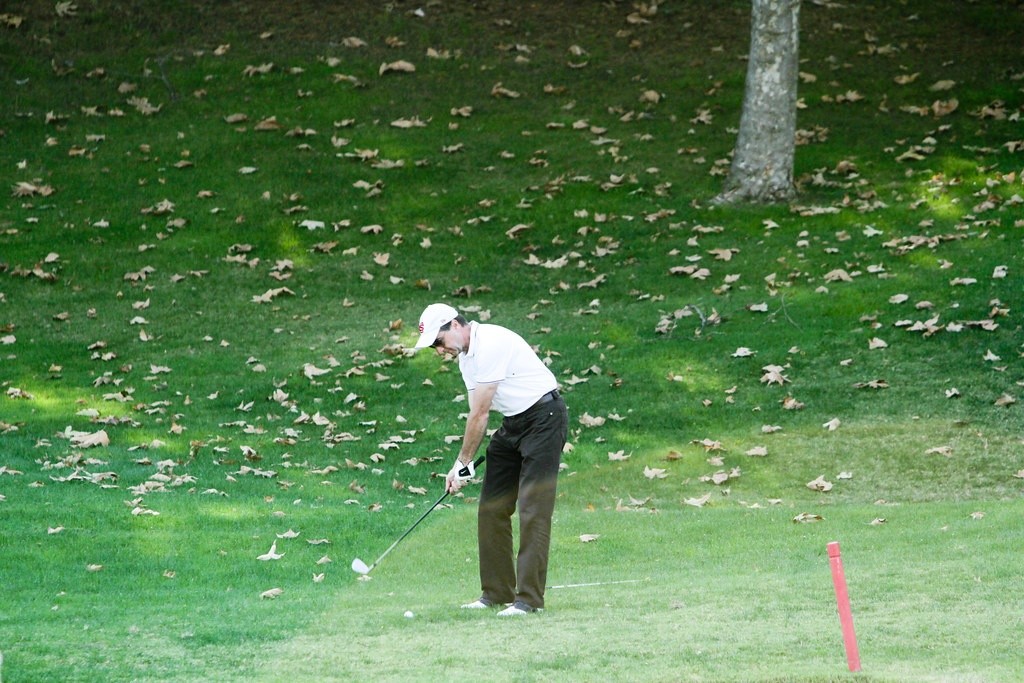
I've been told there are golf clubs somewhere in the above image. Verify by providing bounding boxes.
[351,455,485,574]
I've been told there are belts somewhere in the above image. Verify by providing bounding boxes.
[537,390,559,404]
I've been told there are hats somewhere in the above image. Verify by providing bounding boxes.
[415,303,458,348]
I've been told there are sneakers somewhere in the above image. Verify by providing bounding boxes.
[497,606,543,616]
[461,600,513,609]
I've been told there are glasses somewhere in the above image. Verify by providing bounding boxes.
[429,333,445,349]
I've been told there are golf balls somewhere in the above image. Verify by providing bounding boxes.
[404,611,414,618]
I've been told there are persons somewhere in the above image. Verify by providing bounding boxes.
[415,304,568,616]
